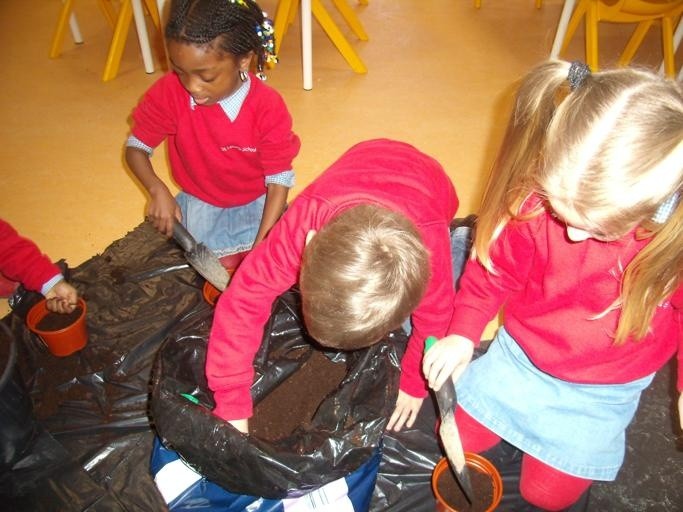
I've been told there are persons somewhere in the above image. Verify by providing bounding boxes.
[0,219,79,314]
[415,60,683,511]
[204,138,459,436]
[122,0,302,273]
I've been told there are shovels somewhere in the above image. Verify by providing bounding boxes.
[174,217,231,292]
[424,335,476,506]
